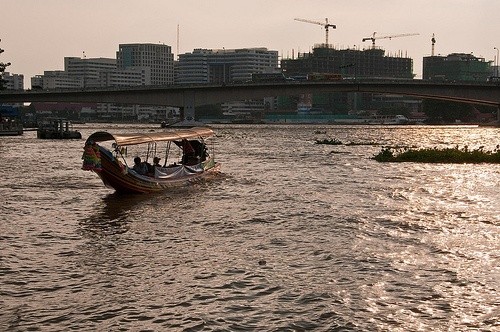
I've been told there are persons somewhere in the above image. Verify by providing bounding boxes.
[153,157,162,168]
[132,157,148,175]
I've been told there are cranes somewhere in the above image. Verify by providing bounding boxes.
[431,33,436,56]
[362,31,420,49]
[293,16,338,73]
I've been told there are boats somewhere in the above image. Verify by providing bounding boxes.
[160,114,205,129]
[0,117,25,136]
[81,125,222,198]
[390,114,409,124]
[36,116,82,140]
[296,107,333,115]
